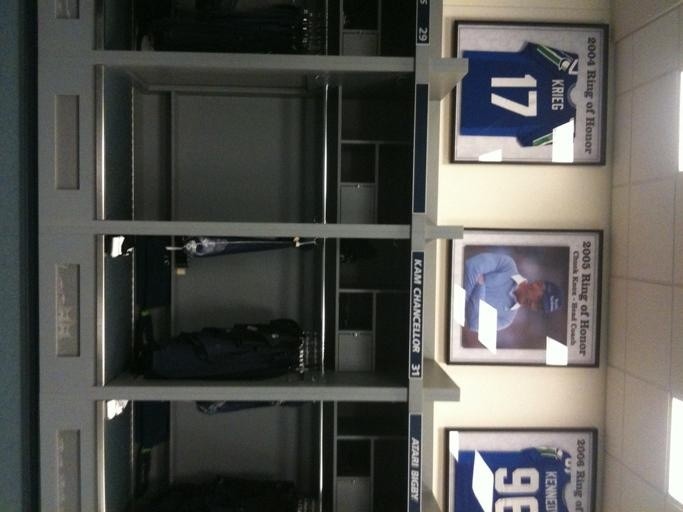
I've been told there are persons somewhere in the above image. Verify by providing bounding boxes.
[465,253,562,334]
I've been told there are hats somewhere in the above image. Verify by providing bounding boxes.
[542,281,562,316]
